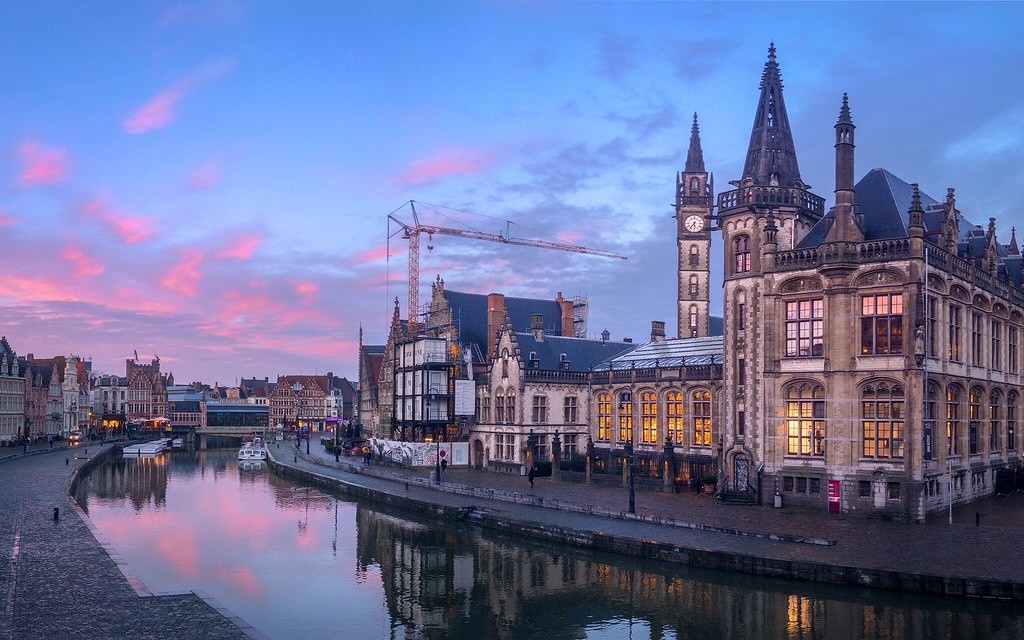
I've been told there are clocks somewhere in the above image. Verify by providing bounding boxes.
[684,213,704,233]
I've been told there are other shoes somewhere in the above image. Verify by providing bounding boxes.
[531,483,533,487]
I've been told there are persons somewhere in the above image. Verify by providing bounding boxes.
[441,459,447,473]
[527,467,534,487]
[50,438,53,448]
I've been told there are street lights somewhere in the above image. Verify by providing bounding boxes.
[89,412,92,426]
[425,388,441,485]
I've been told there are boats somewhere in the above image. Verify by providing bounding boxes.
[149,437,171,445]
[238,438,268,460]
[122,444,162,454]
[172,438,183,445]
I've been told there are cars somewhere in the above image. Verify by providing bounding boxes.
[69,432,83,442]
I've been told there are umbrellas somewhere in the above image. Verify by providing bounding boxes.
[133,417,151,424]
[152,417,170,424]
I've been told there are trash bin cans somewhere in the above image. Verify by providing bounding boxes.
[774,492,782,507]
[430,471,436,480]
[520,466,527,476]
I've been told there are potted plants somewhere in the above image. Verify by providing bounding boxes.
[703,475,718,494]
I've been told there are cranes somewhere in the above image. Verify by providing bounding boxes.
[388,199,628,336]
